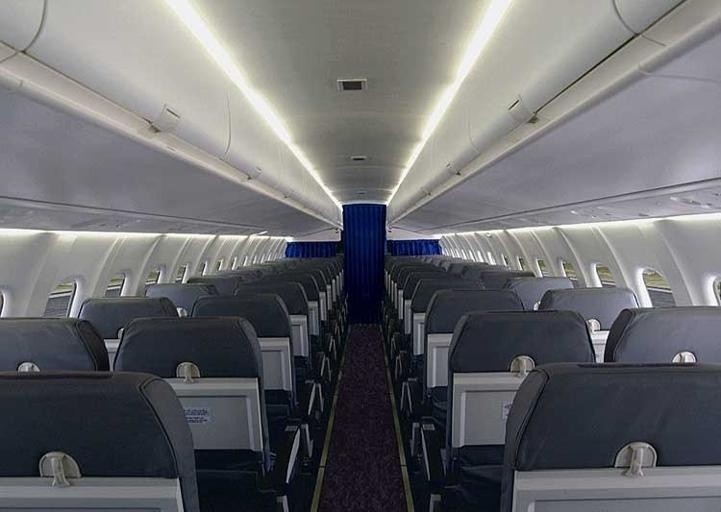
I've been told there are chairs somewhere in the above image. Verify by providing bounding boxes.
[381,255,721,511]
[0,256,348,508]
